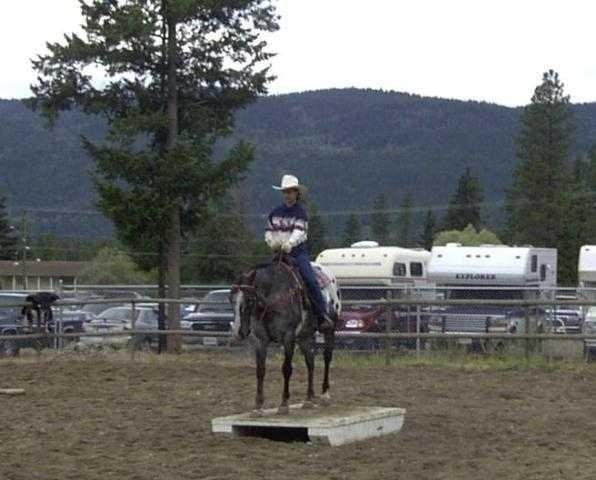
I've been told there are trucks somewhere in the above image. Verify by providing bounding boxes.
[414,240,560,357]
[308,241,435,354]
[577,243,596,364]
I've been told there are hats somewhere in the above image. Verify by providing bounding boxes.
[272,175,307,192]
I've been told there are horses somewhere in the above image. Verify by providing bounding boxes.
[226,260,342,418]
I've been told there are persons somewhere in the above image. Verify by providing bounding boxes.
[262,173,336,333]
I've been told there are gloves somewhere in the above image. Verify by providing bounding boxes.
[269,239,292,253]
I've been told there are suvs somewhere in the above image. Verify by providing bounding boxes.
[537,292,586,336]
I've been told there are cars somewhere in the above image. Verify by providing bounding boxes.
[1,286,256,358]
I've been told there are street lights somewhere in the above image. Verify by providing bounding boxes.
[12,245,42,290]
[9,224,27,290]
